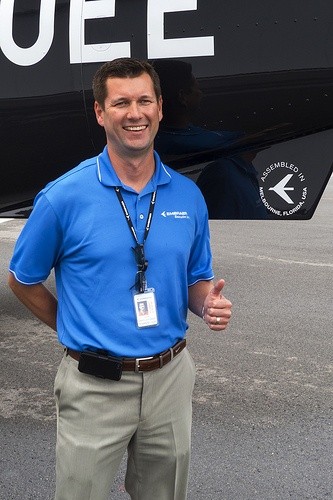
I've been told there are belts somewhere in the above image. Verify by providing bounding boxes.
[66,339,186,372]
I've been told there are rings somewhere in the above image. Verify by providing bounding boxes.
[216,317,221,325]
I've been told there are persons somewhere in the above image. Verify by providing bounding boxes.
[6,58,231,500]
[137,301,148,315]
[145,57,271,220]
